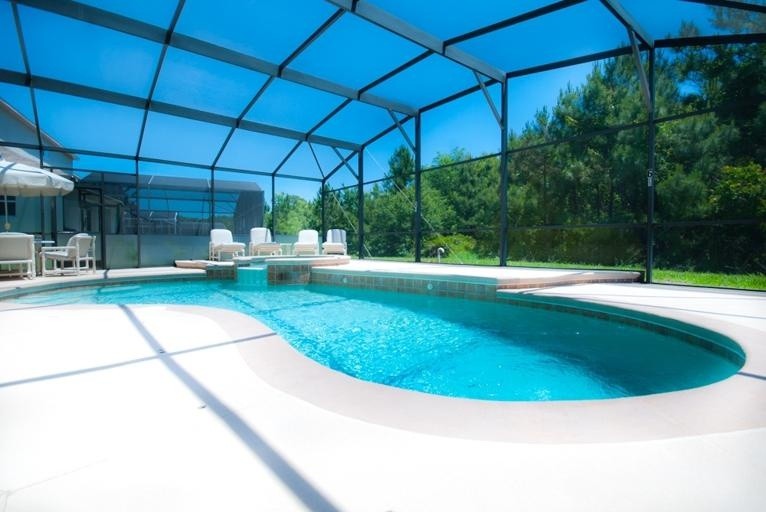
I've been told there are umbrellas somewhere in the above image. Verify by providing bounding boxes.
[0,159,74,231]
[0,146,73,179]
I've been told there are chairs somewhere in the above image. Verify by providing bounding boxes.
[209,227,348,262]
[0,232,97,280]
